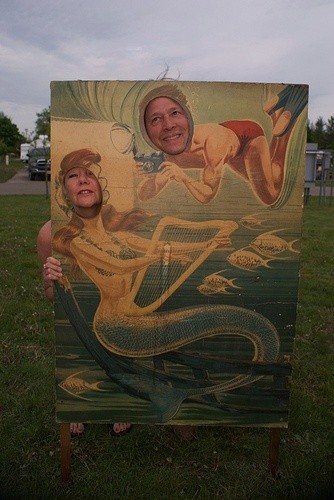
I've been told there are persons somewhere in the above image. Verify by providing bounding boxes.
[36,167,102,303]
[144,96,190,154]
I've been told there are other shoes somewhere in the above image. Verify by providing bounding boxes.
[70,423,86,437]
[111,423,133,437]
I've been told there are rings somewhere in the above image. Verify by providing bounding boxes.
[43,263,47,269]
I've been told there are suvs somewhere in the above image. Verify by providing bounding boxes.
[27,148,51,181]
